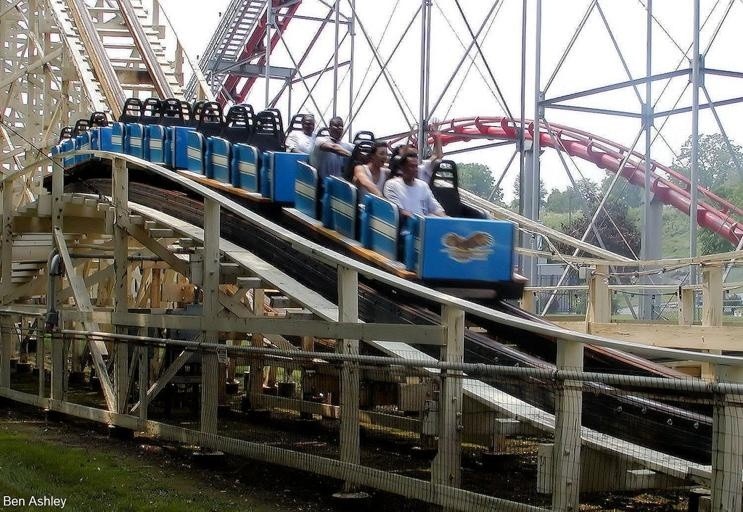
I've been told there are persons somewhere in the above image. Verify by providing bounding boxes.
[391,117,442,184]
[352,140,391,203]
[309,116,356,179]
[284,114,317,155]
[383,152,448,217]
